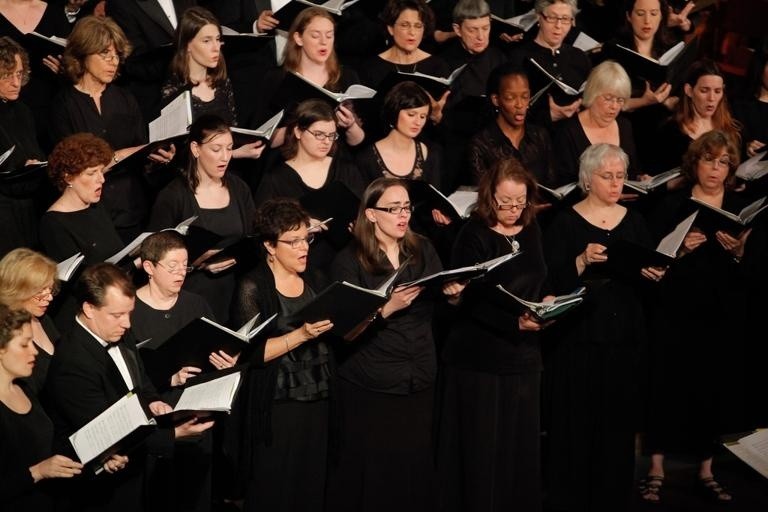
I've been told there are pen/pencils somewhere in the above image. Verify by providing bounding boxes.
[94,467,104,476]
[307,217,333,230]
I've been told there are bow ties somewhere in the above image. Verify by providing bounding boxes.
[104,342,119,349]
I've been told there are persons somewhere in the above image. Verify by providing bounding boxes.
[1,0,768,512]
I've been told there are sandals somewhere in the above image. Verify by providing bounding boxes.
[698,476,733,502]
[639,473,666,503]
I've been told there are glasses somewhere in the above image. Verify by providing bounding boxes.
[304,127,339,141]
[492,194,531,210]
[593,172,629,181]
[277,234,315,248]
[373,205,415,214]
[33,288,59,301]
[157,262,194,274]
[541,12,573,24]
